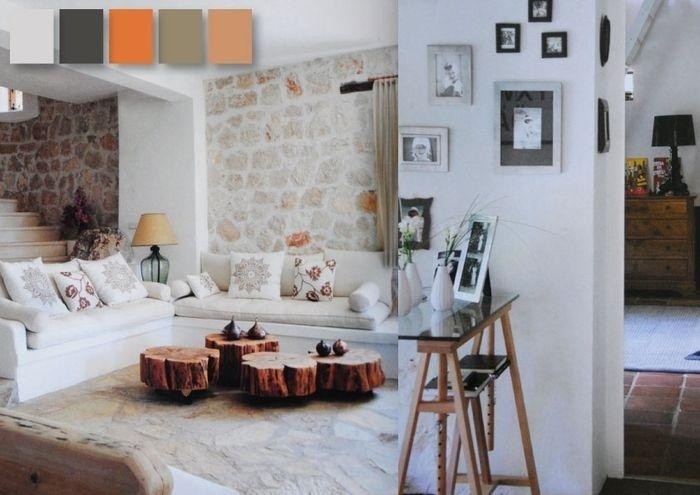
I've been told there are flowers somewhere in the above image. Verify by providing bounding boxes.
[58,186,125,261]
[398,215,417,261]
[440,212,478,265]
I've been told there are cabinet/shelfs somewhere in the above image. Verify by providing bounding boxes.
[626,194,698,298]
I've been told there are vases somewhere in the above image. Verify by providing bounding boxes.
[398,269,411,318]
[431,264,453,310]
[406,261,424,305]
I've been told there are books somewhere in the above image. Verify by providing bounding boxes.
[625,157,649,197]
[422,353,507,399]
[654,158,681,197]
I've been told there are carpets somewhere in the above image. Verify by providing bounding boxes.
[623,304,700,375]
[7,350,400,495]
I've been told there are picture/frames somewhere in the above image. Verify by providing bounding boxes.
[396,126,449,173]
[542,32,567,58]
[600,13,613,67]
[495,23,520,53]
[451,213,499,304]
[494,81,561,173]
[427,44,472,106]
[528,0,552,22]
[397,198,434,249]
[596,98,610,152]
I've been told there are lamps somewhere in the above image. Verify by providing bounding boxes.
[132,212,178,284]
[651,115,695,197]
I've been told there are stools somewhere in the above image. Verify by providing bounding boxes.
[399,289,541,495]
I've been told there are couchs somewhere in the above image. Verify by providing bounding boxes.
[174,251,393,331]
[0,280,174,380]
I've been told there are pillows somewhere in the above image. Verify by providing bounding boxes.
[291,257,337,302]
[53,268,105,314]
[186,271,221,300]
[227,251,283,301]
[0,256,70,317]
[76,251,148,308]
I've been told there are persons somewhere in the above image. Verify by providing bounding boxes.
[533,1,548,17]
[469,263,479,291]
[401,208,423,243]
[412,137,433,162]
[477,230,484,252]
[438,64,464,98]
